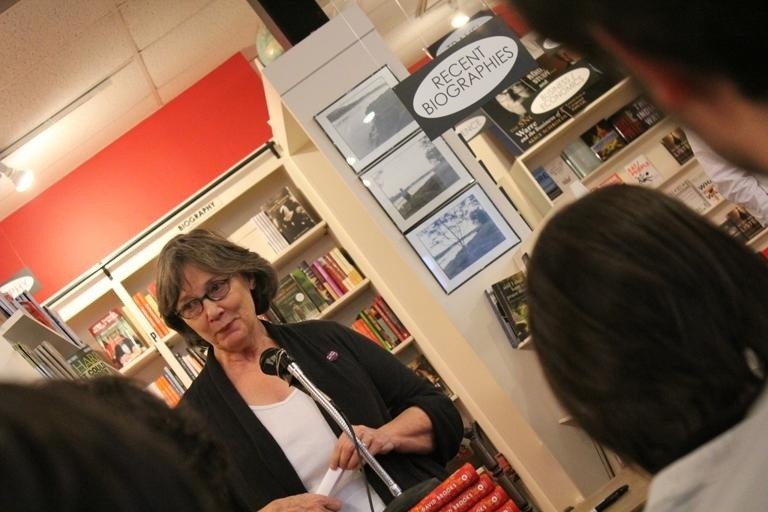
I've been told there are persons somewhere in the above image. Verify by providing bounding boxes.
[525,184,768,512]
[681,127,767,228]
[153,228,464,512]
[512,0,768,175]
[0,372,234,512]
[400,188,411,201]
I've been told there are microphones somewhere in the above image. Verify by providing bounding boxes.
[259,346,333,404]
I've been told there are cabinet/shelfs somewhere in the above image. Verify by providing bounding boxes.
[0,142,560,512]
[515,69,767,258]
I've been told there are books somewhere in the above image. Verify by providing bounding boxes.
[406,462,521,512]
[594,170,626,191]
[716,218,748,243]
[483,285,532,348]
[688,168,723,206]
[175,343,209,381]
[727,205,763,240]
[132,278,173,339]
[490,270,532,338]
[251,185,317,252]
[404,354,454,396]
[148,364,186,409]
[262,247,364,323]
[622,154,663,190]
[0,290,83,346]
[350,296,411,353]
[659,127,694,165]
[10,338,112,382]
[88,305,148,370]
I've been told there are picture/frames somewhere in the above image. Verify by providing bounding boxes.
[404,182,520,295]
[313,64,421,174]
[357,129,476,236]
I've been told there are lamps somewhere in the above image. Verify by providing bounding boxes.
[0,161,37,194]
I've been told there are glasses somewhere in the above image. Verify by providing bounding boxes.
[175,271,236,320]
